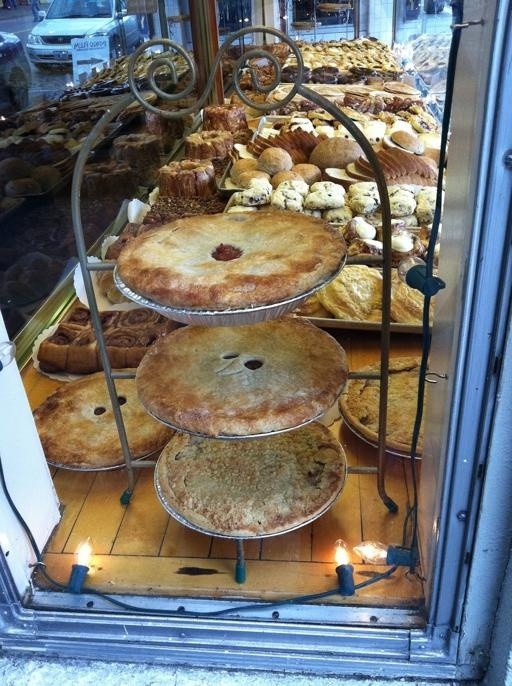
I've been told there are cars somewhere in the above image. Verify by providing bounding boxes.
[0,28,32,114]
[28,0,143,70]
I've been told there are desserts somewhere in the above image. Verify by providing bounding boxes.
[36,306,162,374]
[225,177,445,264]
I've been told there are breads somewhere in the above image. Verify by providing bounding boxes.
[158,421,346,536]
[96,201,230,304]
[137,314,349,436]
[34,369,176,467]
[339,357,432,454]
[298,264,438,325]
[117,211,346,308]
[2,46,239,224]
[122,35,448,199]
